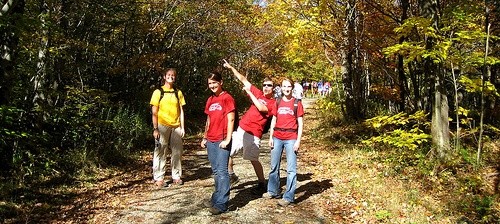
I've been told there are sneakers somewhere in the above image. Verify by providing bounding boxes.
[229,172,239,189]
[263,191,281,199]
[212,207,222,214]
[171,178,183,185]
[155,180,164,186]
[254,180,267,192]
[203,199,214,207]
[277,197,292,207]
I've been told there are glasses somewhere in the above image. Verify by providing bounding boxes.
[262,84,273,87]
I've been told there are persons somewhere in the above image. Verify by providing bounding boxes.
[149,67,186,187]
[272,76,332,102]
[223,59,275,194]
[263,78,304,204]
[200,71,240,214]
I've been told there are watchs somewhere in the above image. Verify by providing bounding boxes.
[153,128,158,130]
